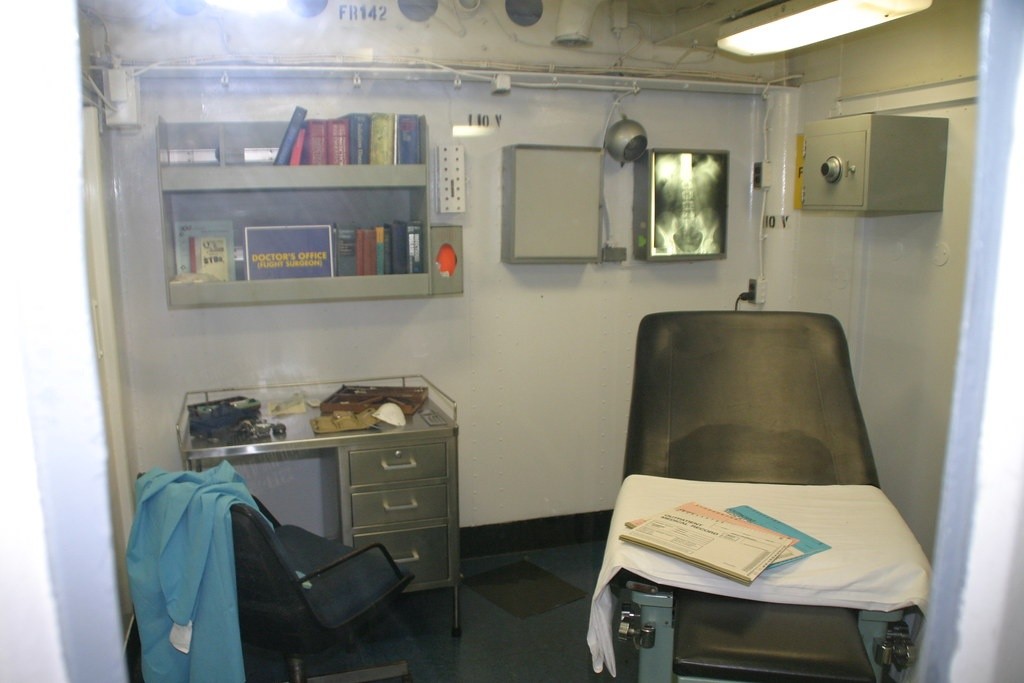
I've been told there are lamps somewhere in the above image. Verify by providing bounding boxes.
[600,119,648,262]
[717,0,932,57]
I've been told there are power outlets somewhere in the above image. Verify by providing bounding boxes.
[749,277,766,304]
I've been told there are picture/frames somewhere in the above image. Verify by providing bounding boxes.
[242,223,336,280]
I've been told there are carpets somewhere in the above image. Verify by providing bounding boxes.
[463,560,589,621]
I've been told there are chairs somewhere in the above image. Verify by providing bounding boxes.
[125,460,416,683]
[585,311,932,683]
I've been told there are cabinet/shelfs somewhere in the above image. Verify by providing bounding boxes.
[176,374,461,640]
[155,114,431,313]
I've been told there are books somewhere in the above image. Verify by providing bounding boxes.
[335,218,423,277]
[273,104,420,165]
